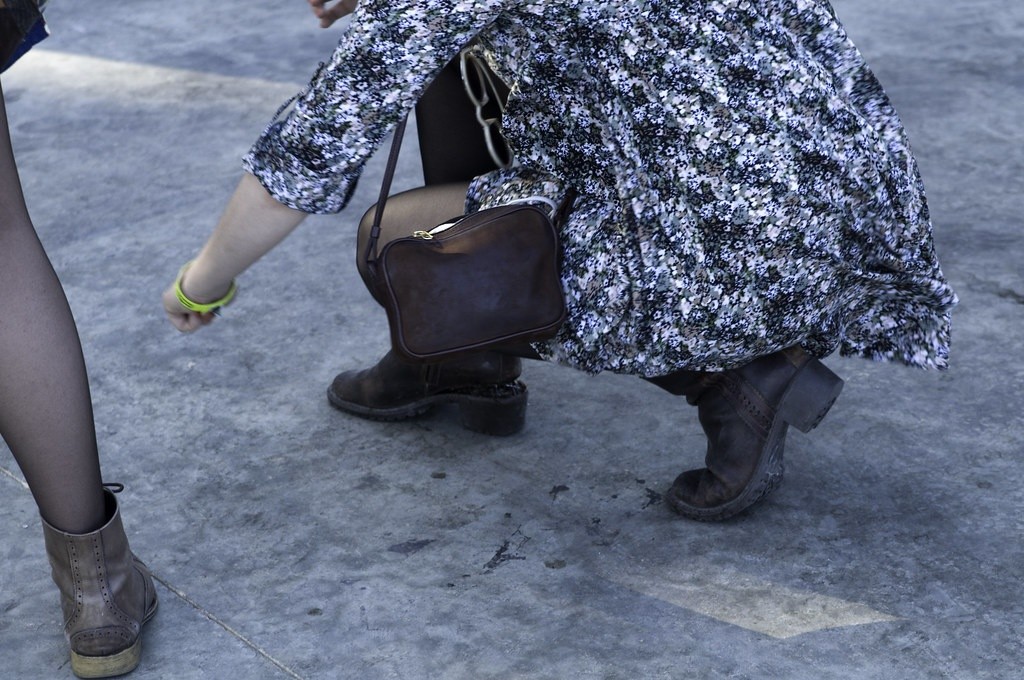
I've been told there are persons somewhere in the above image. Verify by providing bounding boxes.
[160,0,961,524]
[0,0,159,679]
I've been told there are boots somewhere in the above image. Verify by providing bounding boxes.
[38,479,157,679]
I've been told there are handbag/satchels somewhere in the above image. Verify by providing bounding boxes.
[376,205,568,363]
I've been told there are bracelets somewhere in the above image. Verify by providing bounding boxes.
[176,259,239,314]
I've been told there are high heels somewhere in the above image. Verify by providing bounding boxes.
[327,330,530,436]
[668,337,847,524]
